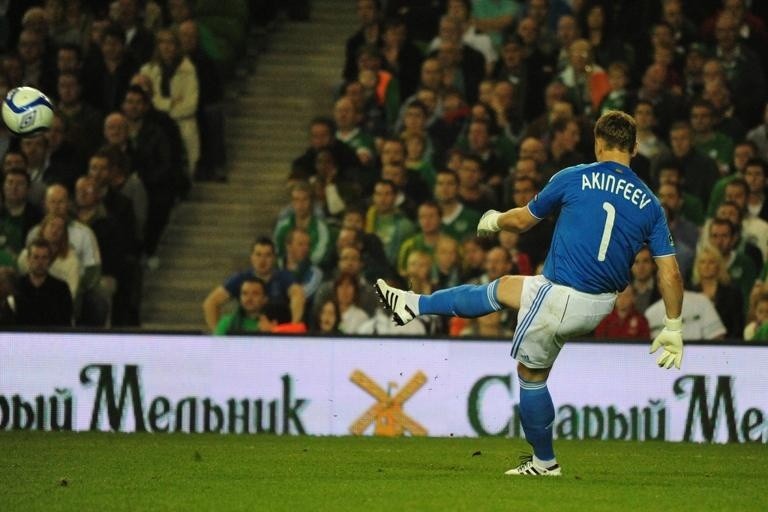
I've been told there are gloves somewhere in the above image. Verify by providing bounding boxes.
[648,315,684,371]
[475,208,502,239]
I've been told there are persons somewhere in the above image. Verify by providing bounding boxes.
[373,111,685,476]
[1,0,260,335]
[204,1,767,347]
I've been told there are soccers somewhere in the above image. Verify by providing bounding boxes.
[2,86,52,136]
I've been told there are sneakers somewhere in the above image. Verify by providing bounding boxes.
[374,277,419,328]
[504,451,563,478]
